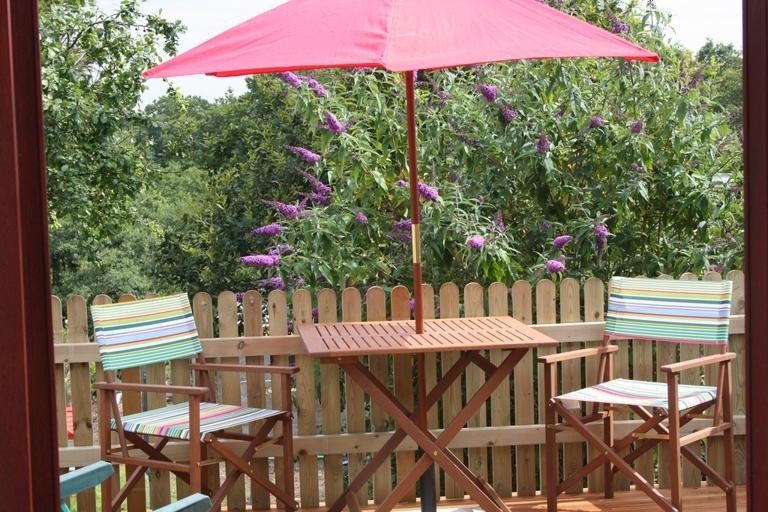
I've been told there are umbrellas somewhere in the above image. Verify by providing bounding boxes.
[134,0,663,511]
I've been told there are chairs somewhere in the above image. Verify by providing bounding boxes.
[89,293,299,511]
[58,461,212,511]
[537,275,737,512]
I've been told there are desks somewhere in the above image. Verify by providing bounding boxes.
[297,315,559,512]
